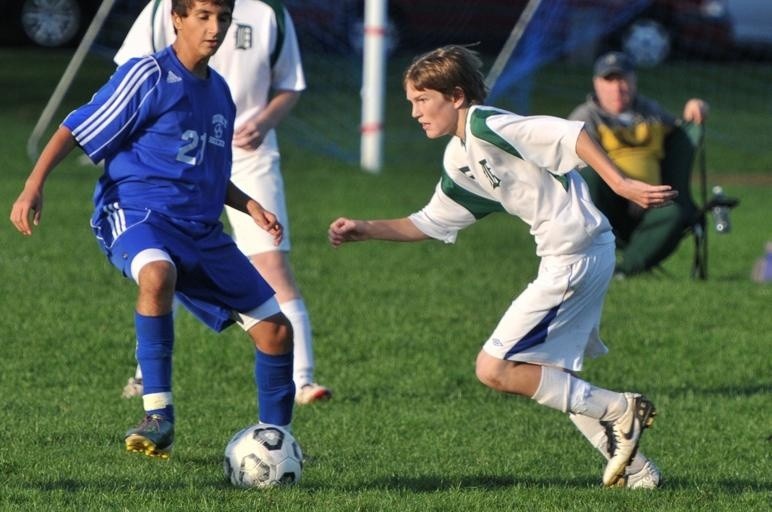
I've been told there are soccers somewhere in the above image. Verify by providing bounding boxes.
[223,424,304,489]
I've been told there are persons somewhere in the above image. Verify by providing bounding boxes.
[8,0,294,459]
[566,52,710,276]
[114,0,332,405]
[330,42,680,491]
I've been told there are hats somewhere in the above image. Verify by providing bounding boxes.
[593,51,636,76]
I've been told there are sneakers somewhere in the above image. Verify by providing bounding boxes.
[294,382,332,405]
[122,377,174,459]
[602,392,661,489]
